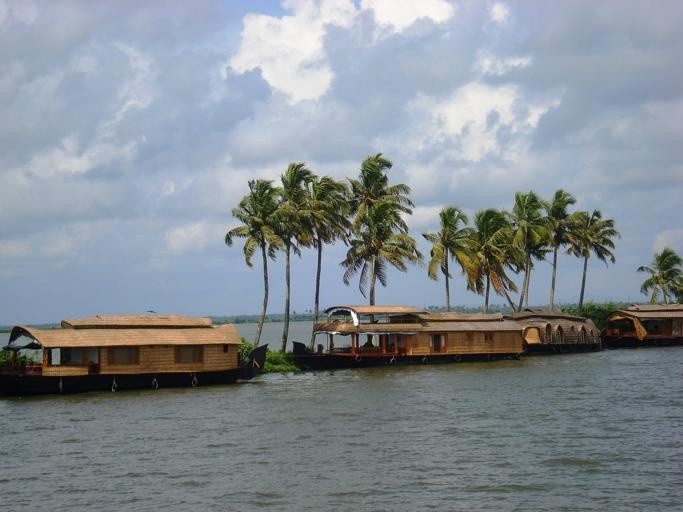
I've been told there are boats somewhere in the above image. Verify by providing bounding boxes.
[289,305,525,370]
[0,315,269,394]
[503,308,604,352]
[604,303,683,345]
